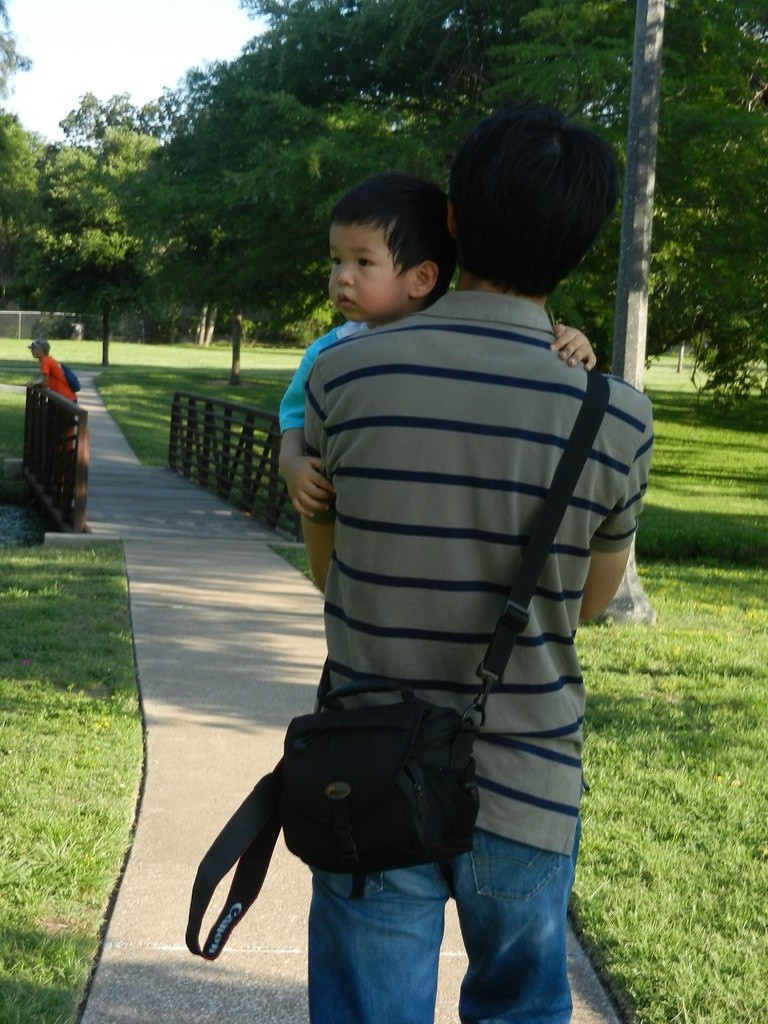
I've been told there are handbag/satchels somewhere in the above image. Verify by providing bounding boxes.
[279,677,480,877]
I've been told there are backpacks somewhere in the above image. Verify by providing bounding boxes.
[61,363,81,392]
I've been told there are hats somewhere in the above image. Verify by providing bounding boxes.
[28,338,51,353]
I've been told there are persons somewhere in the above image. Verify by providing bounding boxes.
[27,339,78,485]
[278,173,598,518]
[300,104,655,1024]
